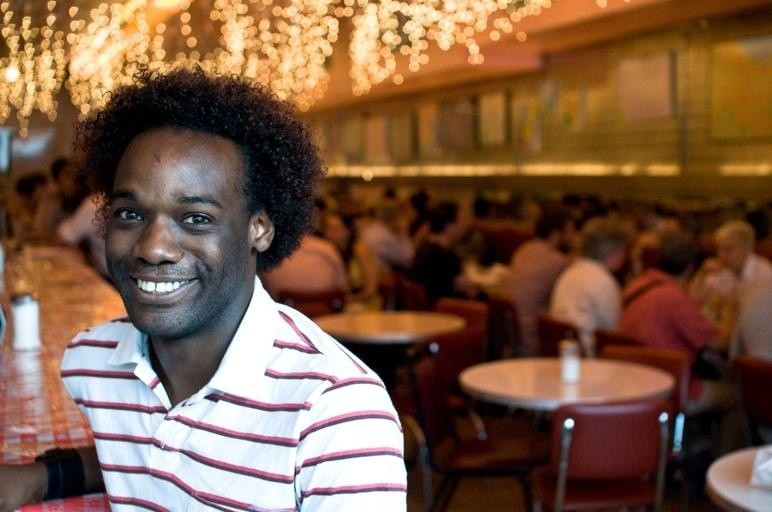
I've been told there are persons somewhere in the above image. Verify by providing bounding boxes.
[29,156,76,241]
[0,55,408,512]
[402,175,772,473]
[263,182,428,293]
[10,173,49,243]
[55,189,110,279]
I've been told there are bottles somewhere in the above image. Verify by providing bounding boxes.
[11,291,42,352]
[560,339,579,385]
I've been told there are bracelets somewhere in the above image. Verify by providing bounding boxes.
[32,447,86,503]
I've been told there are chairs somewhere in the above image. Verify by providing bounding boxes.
[258,225,772,440]
[403,338,538,512]
[539,396,683,512]
[396,410,433,511]
